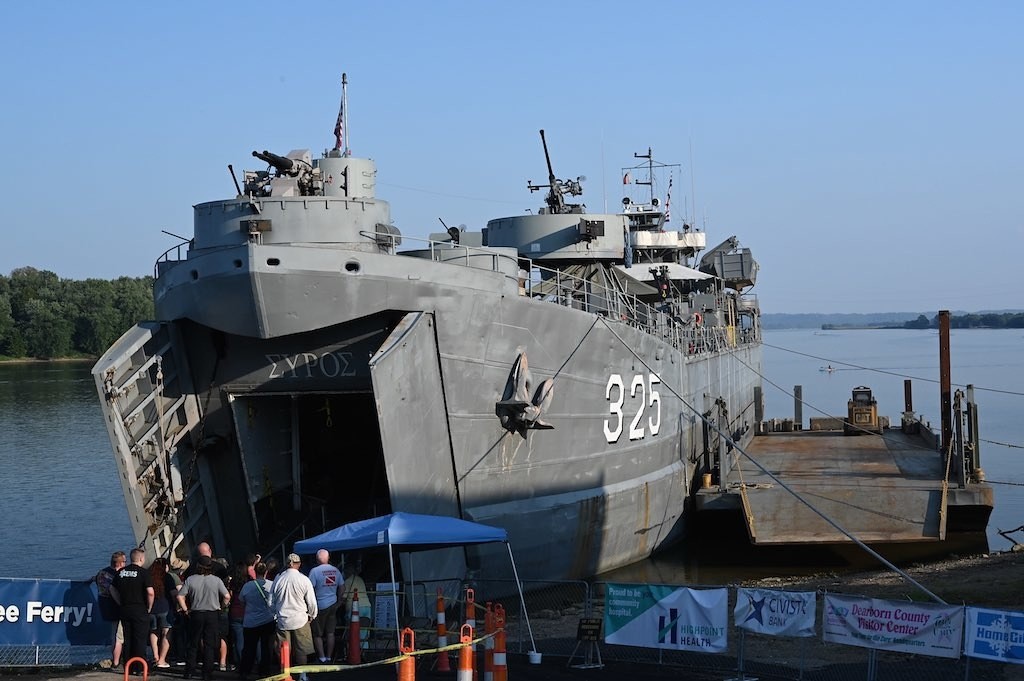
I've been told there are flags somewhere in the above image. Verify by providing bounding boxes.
[334,94,344,150]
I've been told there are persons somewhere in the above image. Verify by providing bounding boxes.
[92,541,370,681]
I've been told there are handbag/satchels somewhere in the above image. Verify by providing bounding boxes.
[271,620,276,635]
[336,602,347,619]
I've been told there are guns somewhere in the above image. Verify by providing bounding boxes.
[539,129,565,209]
[527,176,583,197]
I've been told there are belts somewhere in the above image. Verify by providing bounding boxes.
[192,610,213,612]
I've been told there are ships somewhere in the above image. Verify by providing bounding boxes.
[90,70,767,635]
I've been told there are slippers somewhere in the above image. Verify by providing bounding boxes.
[231,665,236,670]
[220,665,227,671]
[157,661,170,667]
[153,661,158,667]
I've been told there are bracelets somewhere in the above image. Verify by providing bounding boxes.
[184,609,188,613]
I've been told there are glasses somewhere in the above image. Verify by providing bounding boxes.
[119,560,127,563]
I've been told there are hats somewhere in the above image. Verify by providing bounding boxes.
[287,553,300,566]
[199,556,212,566]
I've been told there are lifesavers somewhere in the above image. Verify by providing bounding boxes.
[693,313,701,324]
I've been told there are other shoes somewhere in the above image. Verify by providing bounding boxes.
[327,660,331,665]
[176,660,186,665]
[198,661,203,665]
[137,671,149,676]
[313,659,326,665]
[203,674,217,681]
[183,672,192,679]
[214,661,219,665]
[299,676,308,681]
[111,664,124,670]
[133,670,138,674]
